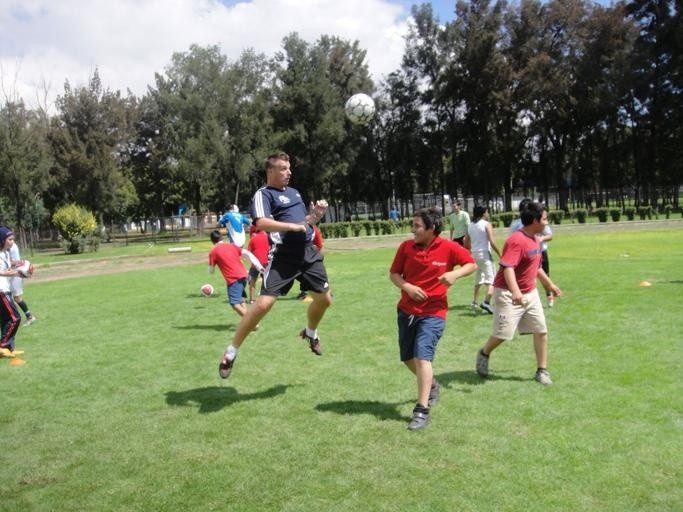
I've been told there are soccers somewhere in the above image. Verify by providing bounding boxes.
[201,285,213,296]
[345,94,375,123]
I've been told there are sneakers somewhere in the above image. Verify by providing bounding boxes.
[297,292,308,299]
[471,302,493,314]
[299,328,322,356]
[429,378,440,407]
[408,402,430,431]
[476,349,489,378]
[546,292,553,308]
[220,348,237,379]
[534,370,552,385]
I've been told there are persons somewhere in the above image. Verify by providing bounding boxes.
[0,223,34,359]
[450,200,472,249]
[507,199,555,308]
[217,150,334,380]
[474,202,562,386]
[463,205,501,315]
[389,206,399,221]
[388,206,478,431]
[5,242,36,328]
[203,203,326,330]
